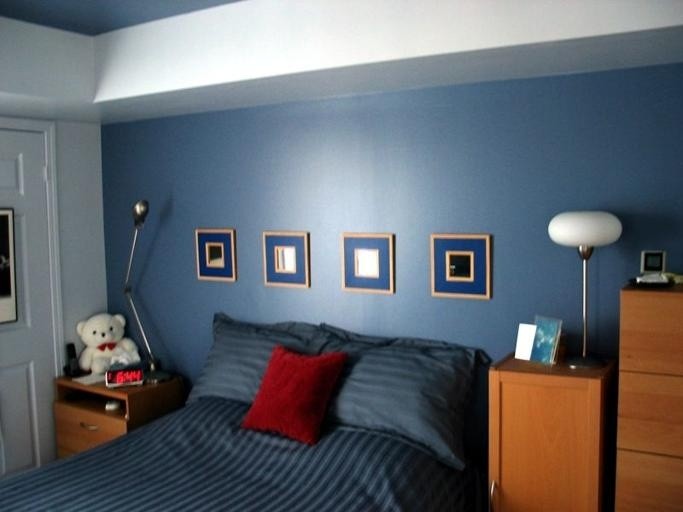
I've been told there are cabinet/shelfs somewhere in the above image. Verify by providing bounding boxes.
[618,275,681,512]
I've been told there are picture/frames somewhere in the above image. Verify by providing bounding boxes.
[261,230,310,288]
[0,208,18,324]
[340,233,395,294]
[430,233,490,301]
[195,229,236,282]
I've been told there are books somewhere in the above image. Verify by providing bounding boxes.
[104,368,144,388]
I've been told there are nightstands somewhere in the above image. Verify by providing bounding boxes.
[490,354,617,511]
[53,367,184,460]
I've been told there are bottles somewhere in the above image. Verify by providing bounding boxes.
[67,342,79,375]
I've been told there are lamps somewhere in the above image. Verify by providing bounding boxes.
[547,212,622,369]
[123,199,174,383]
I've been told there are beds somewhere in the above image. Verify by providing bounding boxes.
[0,326,478,512]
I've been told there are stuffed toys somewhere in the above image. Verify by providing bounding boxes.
[75,312,144,376]
